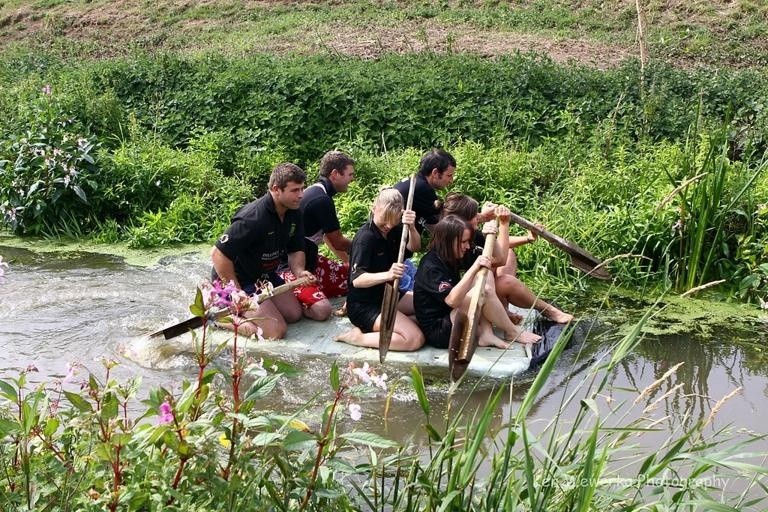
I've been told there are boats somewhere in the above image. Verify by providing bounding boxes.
[176,261,538,381]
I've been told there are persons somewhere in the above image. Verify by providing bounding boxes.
[203,162,318,343]
[434,189,577,324]
[275,150,358,323]
[390,147,458,291]
[331,187,426,352]
[412,215,546,350]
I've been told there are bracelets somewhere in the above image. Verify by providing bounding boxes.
[527,234,533,245]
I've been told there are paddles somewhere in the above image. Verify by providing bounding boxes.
[379,175,417,364]
[449,220,496,383]
[143,277,309,340]
[510,212,612,280]
